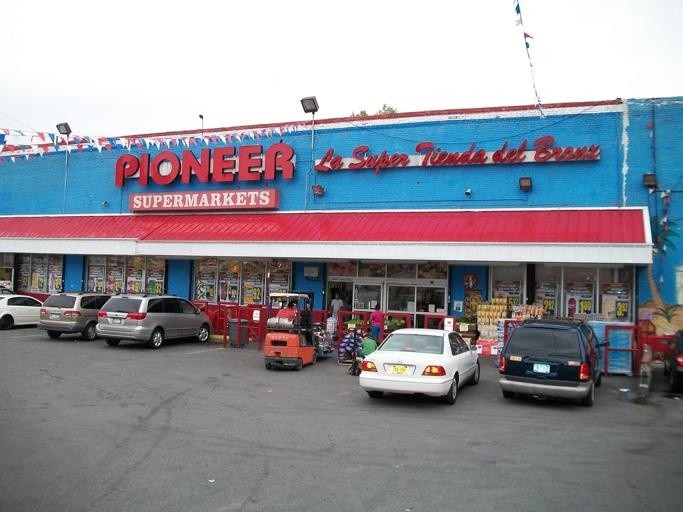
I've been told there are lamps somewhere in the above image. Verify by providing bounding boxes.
[518,177,532,191]
[55,121,71,144]
[642,172,656,188]
[311,183,324,197]
[300,95,320,160]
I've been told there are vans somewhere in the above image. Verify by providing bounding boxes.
[96,291,214,348]
[39,292,111,341]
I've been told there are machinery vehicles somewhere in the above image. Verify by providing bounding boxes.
[263,292,335,371]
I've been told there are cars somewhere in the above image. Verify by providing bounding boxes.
[661,328,683,393]
[357,327,479,404]
[0,285,17,299]
[0,295,43,330]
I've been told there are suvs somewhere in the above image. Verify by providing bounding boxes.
[497,312,610,407]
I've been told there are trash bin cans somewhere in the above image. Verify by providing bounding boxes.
[228,318,248,348]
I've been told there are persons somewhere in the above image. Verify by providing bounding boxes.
[275,302,296,320]
[367,304,384,343]
[329,292,344,318]
[352,333,377,374]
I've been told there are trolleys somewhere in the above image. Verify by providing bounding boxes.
[348,326,362,376]
